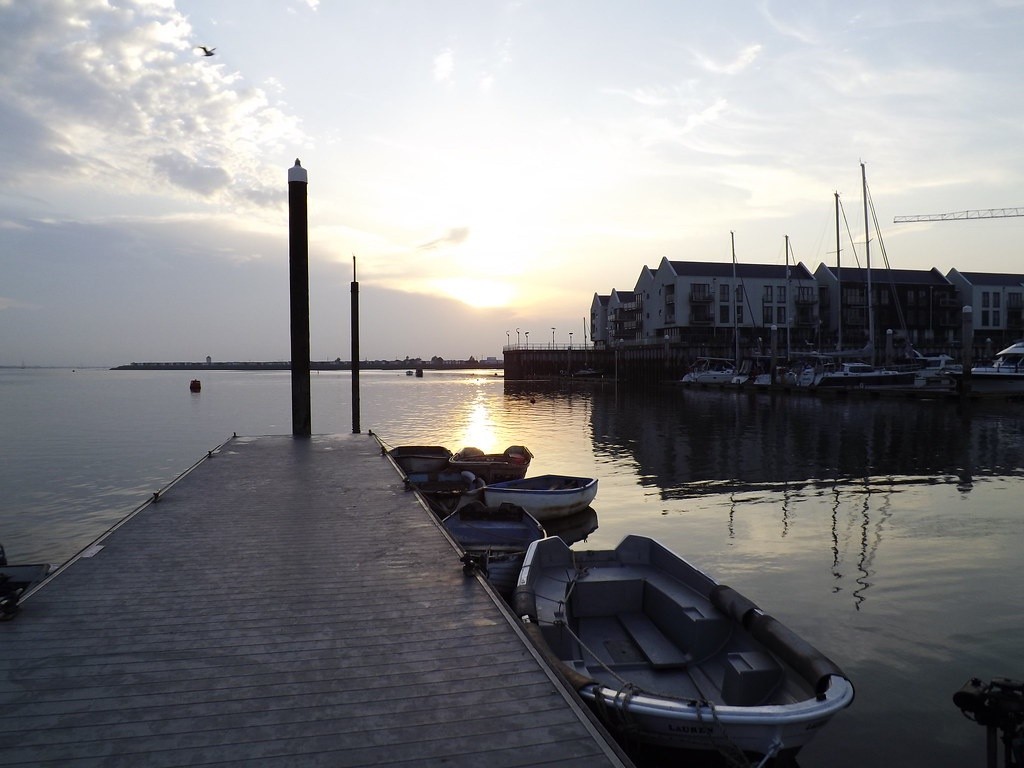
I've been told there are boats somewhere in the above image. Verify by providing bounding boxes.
[913,348,961,375]
[449,446,534,481]
[514,536,855,762]
[416,366,423,377]
[410,465,485,504]
[388,445,453,470]
[682,357,734,383]
[406,371,414,375]
[970,340,1024,398]
[481,473,598,516]
[439,501,547,592]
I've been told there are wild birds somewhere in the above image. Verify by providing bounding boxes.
[198,45,216,56]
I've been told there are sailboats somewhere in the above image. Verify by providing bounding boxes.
[723,160,928,388]
